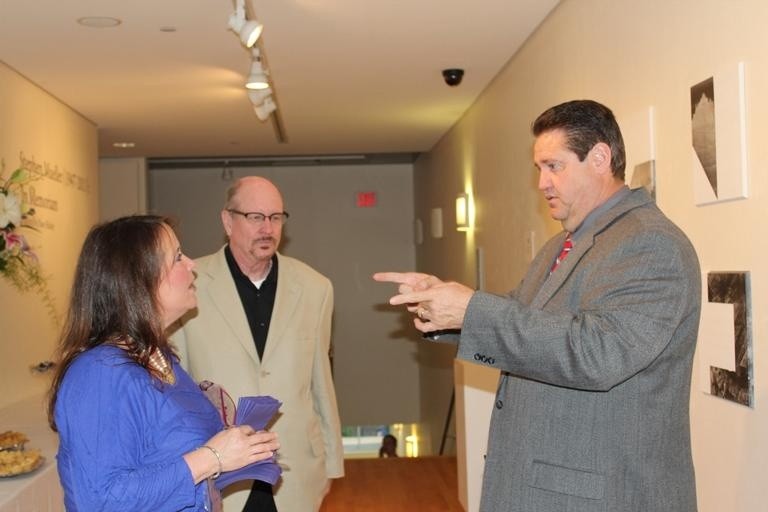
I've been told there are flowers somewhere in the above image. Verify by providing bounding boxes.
[0,166,62,328]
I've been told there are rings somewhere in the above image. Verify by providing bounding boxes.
[420,310,426,318]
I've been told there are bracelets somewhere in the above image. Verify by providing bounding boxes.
[196,444,223,479]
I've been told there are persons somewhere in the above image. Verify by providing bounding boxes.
[164,177,346,512]
[371,101,702,512]
[41,214,282,512]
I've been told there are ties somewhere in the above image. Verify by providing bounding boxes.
[549,232,572,274]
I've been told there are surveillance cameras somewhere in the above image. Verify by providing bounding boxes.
[442,68,463,86]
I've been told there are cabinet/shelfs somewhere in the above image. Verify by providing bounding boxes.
[0,422,65,512]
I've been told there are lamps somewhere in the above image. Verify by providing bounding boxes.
[226,0,286,143]
[455,193,470,232]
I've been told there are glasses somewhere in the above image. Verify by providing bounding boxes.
[228,208,289,224]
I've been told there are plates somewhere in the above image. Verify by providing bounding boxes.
[0,447,46,478]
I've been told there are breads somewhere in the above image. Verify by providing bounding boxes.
[0,431,29,449]
[0,450,39,477]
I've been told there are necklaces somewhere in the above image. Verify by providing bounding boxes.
[142,342,170,376]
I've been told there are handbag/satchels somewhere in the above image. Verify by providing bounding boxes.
[198,380,236,427]
